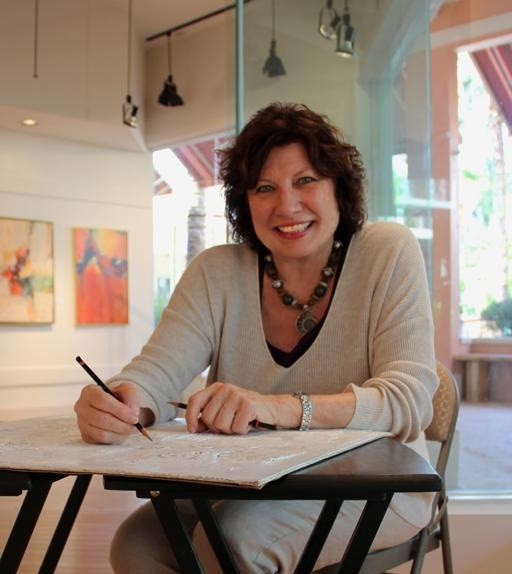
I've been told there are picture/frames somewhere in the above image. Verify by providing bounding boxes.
[1,215,57,326]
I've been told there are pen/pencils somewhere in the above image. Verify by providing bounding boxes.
[76,356,155,443]
[167,400,280,430]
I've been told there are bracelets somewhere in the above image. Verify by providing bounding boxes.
[293,390,313,431]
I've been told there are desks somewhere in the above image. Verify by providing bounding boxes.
[0,434,446,574]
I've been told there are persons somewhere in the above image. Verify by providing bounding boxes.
[75,102,441,574]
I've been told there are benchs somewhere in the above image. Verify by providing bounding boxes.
[458,353,511,403]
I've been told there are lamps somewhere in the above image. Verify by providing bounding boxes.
[334,0,359,59]
[318,0,342,40]
[142,1,285,114]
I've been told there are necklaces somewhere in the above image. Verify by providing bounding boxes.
[263,242,342,336]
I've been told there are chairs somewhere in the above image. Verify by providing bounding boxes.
[319,360,460,574]
